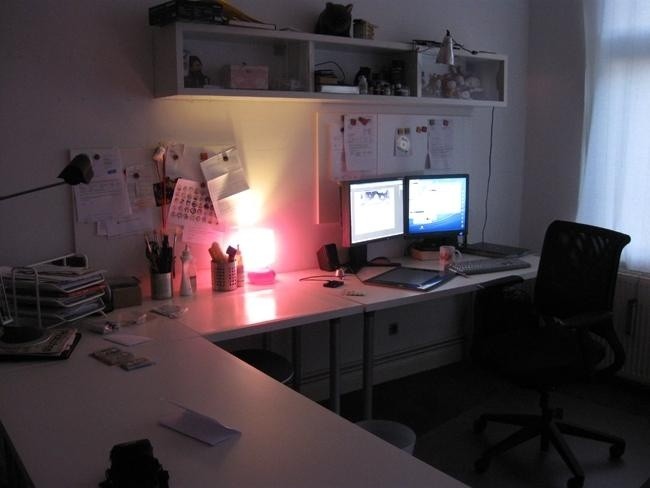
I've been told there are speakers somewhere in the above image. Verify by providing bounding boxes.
[317,243,339,271]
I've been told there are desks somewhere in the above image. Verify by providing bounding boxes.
[363,255,542,421]
[144,271,365,414]
[0,298,475,488]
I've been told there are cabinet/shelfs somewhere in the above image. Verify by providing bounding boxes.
[154,21,512,110]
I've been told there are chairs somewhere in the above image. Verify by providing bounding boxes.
[471,218,631,488]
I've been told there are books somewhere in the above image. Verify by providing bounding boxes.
[2,263,107,332]
[0,323,82,359]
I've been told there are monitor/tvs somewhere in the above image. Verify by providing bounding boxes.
[339,176,407,274]
[405,174,469,252]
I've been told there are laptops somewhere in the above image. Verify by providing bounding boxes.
[514,244,515,245]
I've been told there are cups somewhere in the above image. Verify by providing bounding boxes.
[440,247,461,271]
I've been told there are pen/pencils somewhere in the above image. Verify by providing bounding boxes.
[143,228,179,273]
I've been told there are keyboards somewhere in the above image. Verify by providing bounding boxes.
[443,256,532,275]
[459,241,532,259]
